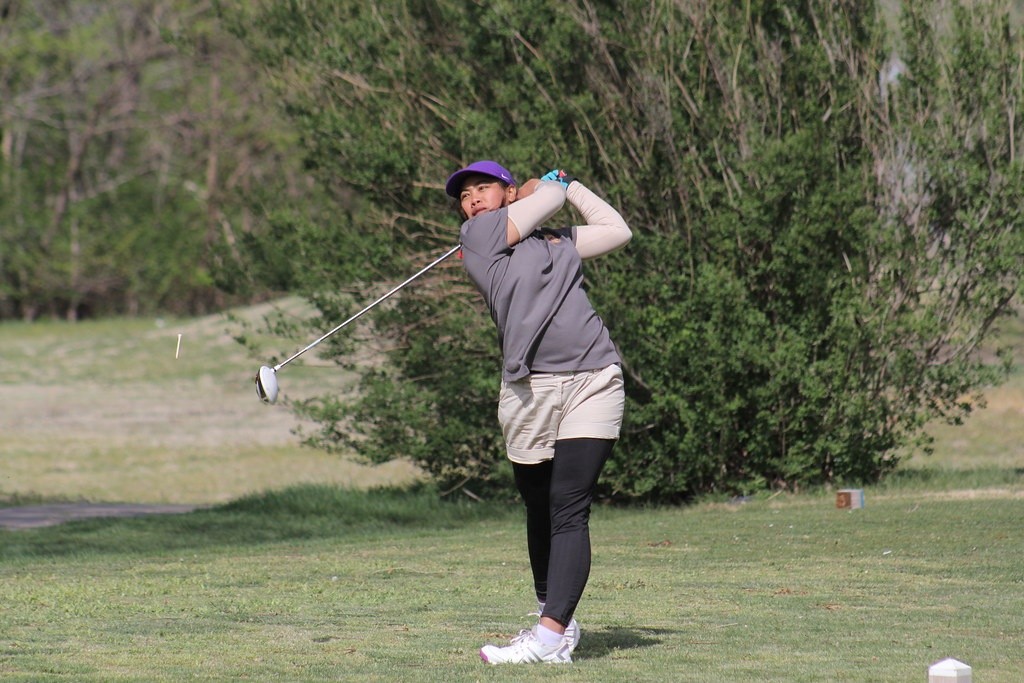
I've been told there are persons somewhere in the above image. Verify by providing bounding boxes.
[445,160,634,663]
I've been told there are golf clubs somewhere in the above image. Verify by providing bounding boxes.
[255,167,569,407]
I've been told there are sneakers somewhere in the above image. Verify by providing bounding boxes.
[480,629,573,665]
[521,606,580,653]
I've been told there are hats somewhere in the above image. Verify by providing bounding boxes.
[446,161,517,199]
